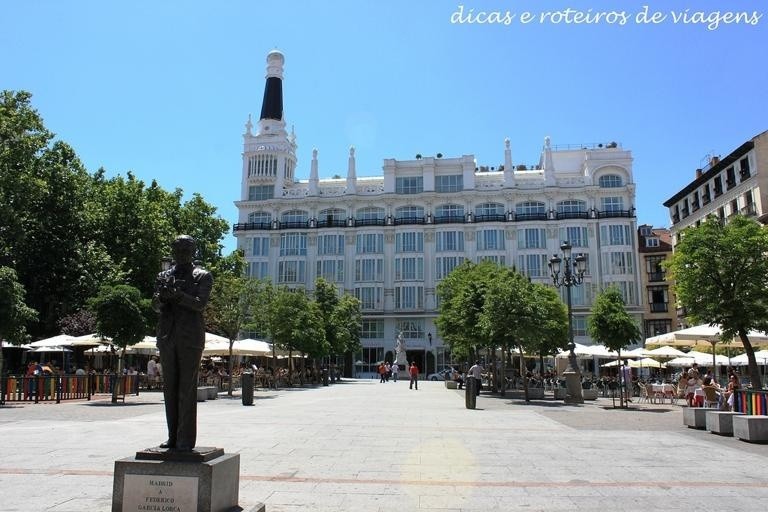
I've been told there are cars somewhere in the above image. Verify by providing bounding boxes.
[427,367,462,381]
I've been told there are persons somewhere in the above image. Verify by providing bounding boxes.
[2,332,309,360]
[150,234,214,455]
[26,355,264,389]
[444,360,743,411]
[378,361,419,390]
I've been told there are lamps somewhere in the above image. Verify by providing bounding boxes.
[428,333,432,344]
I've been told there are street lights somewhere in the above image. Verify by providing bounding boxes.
[548,240,588,405]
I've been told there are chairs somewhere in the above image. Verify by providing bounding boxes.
[638,382,721,409]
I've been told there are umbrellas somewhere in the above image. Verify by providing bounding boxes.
[495,320,768,369]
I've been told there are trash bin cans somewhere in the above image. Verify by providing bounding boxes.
[323,369,328,386]
[465,375,476,409]
[241,372,254,406]
